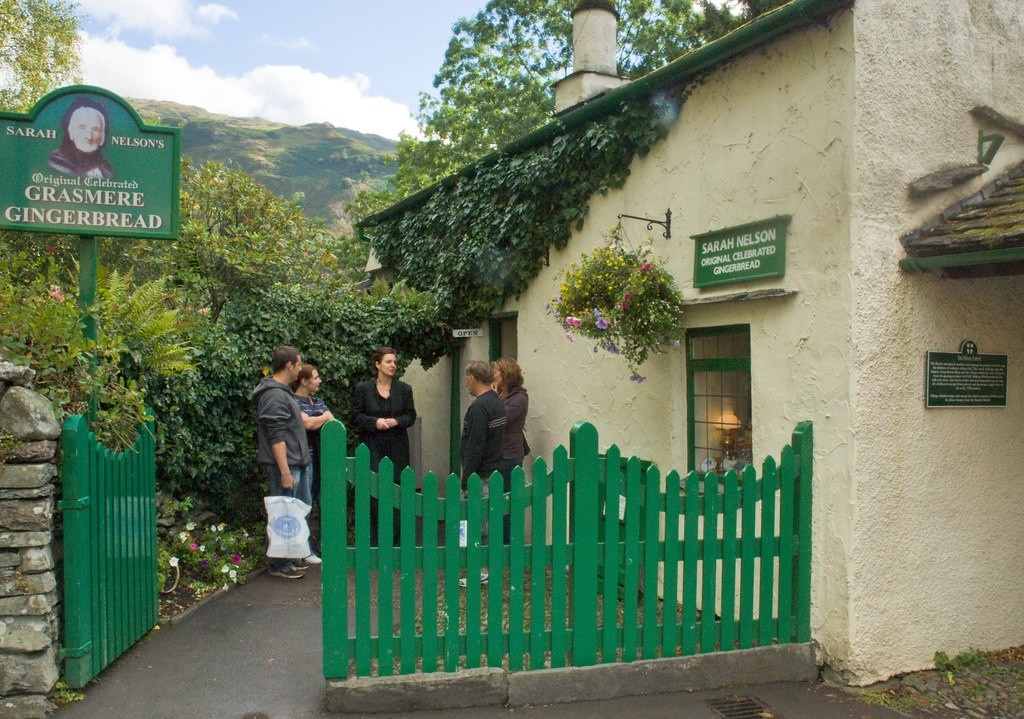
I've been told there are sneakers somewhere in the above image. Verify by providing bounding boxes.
[293,562,309,570]
[269,567,305,579]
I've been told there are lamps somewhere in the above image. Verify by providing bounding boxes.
[716,411,742,468]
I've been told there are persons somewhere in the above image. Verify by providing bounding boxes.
[458,360,507,588]
[294,365,334,565]
[251,347,309,578]
[491,357,531,545]
[352,347,417,547]
[50,97,111,178]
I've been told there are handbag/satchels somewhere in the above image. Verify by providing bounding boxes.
[522,431,531,455]
[458,496,468,547]
[263,487,312,558]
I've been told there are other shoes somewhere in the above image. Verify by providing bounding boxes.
[458,572,489,587]
[304,555,322,564]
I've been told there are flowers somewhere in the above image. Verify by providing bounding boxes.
[548,225,688,384]
